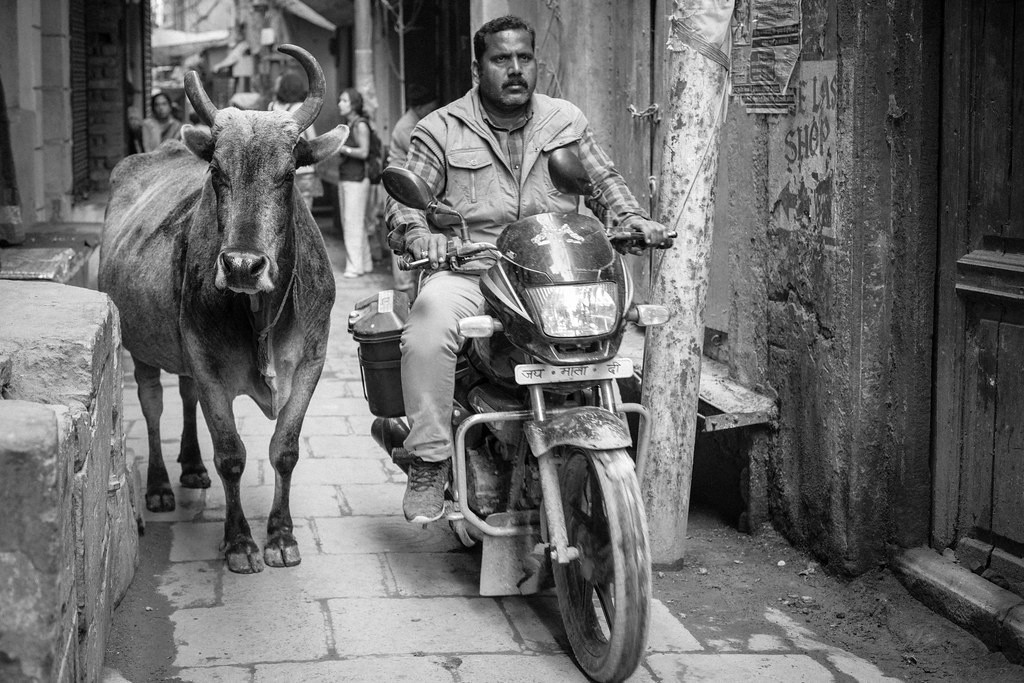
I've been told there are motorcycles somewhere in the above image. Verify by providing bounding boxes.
[347,147,681,683]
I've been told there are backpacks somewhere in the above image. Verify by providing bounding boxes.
[349,116,383,185]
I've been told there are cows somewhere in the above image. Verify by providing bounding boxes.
[97,42,350,575]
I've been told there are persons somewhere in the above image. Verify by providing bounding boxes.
[334,90,374,278]
[384,14,667,525]
[387,83,444,308]
[266,72,325,215]
[139,93,185,155]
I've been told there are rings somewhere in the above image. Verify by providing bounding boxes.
[421,250,428,257]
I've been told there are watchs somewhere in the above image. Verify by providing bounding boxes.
[345,146,352,157]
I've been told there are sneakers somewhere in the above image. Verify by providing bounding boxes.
[402,456,452,523]
